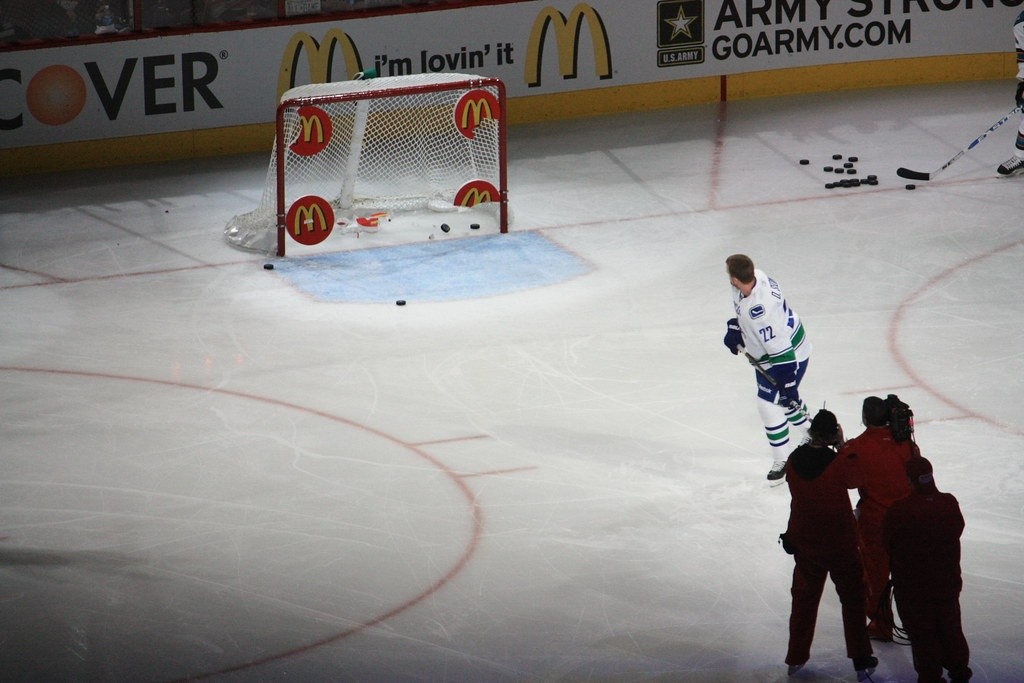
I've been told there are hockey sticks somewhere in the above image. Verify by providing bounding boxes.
[735,343,826,424]
[896,102,1024,181]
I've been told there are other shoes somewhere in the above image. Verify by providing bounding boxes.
[866,621,892,639]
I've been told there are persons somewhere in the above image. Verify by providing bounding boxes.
[997,11,1024,178]
[879,457,973,683]
[782,409,879,671]
[837,395,922,643]
[723,254,812,488]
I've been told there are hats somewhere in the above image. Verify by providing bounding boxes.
[812,409,839,435]
[907,456,935,493]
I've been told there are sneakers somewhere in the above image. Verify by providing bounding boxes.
[767,457,786,487]
[996,155,1024,176]
[785,648,810,675]
[853,656,878,682]
[798,430,810,448]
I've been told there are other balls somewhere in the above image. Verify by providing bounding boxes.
[823,154,879,189]
[799,159,809,165]
[440,222,452,233]
[905,184,916,190]
[469,223,480,230]
[395,300,407,306]
[263,263,274,270]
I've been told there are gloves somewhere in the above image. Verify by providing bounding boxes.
[780,375,799,409]
[724,318,745,355]
[1015,81,1024,113]
